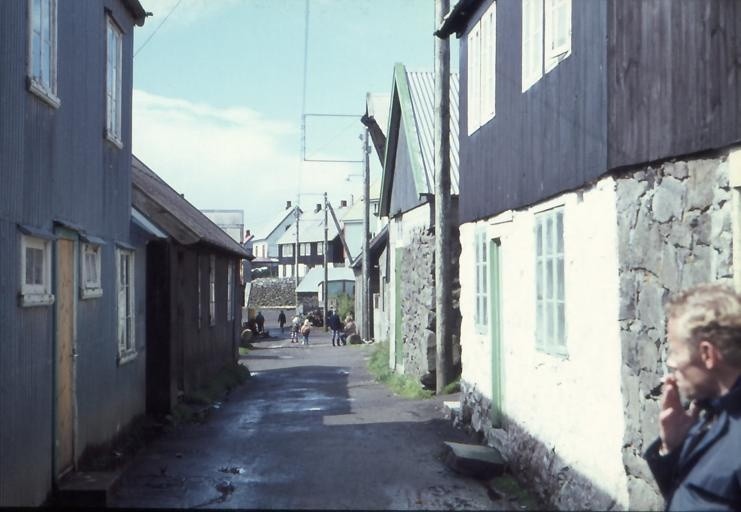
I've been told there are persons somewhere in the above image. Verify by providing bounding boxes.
[256,310,356,346]
[643,283,741,512]
